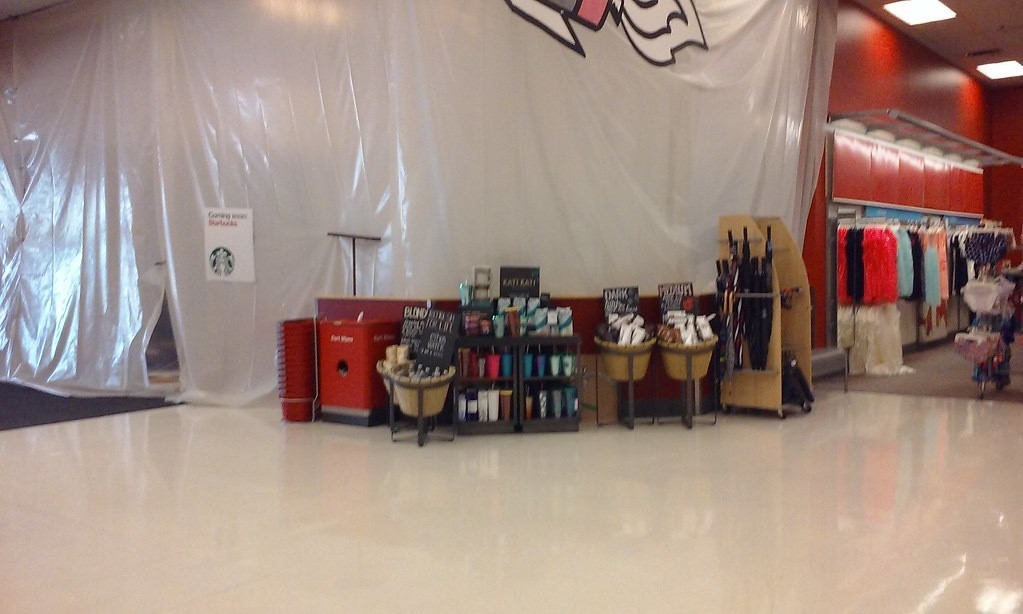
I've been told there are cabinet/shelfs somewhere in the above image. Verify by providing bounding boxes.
[521,336,582,432]
[454,332,519,433]
[714,286,813,417]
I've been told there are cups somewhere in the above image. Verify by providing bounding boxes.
[457,387,512,423]
[505,307,519,339]
[526,386,576,420]
[493,314,508,339]
[459,282,472,308]
[459,348,512,378]
[523,353,573,377]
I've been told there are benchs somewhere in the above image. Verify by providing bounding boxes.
[810,347,849,393]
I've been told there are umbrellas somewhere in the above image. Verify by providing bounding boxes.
[716,225,774,394]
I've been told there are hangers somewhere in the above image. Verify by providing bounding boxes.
[954,266,1014,342]
[838,216,1010,236]
